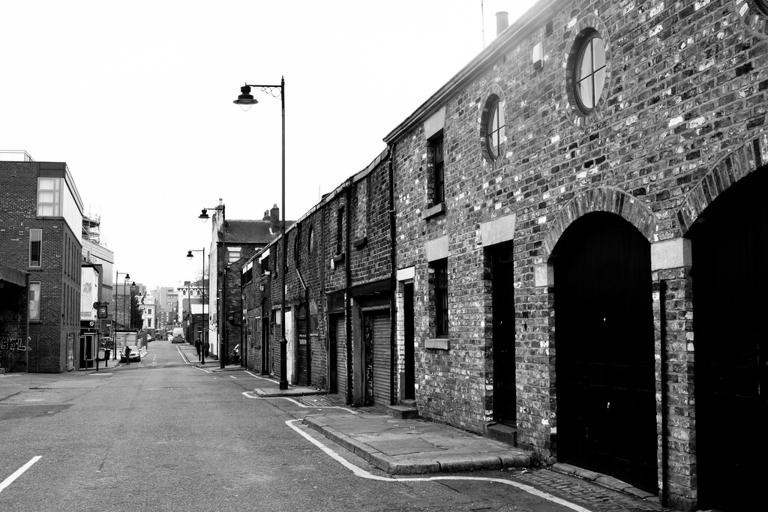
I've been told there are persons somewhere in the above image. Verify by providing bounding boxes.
[194,335,203,355]
[124,345,130,365]
[203,340,210,358]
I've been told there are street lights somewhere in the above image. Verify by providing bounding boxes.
[186,247,207,366]
[198,205,228,370]
[232,74,291,391]
[113,271,147,361]
